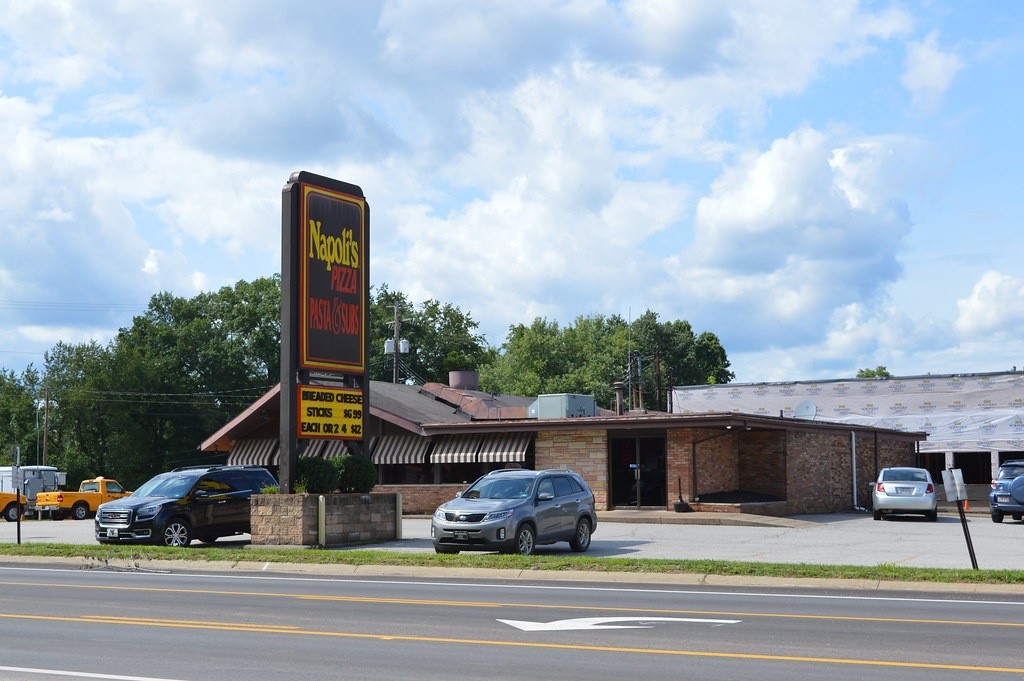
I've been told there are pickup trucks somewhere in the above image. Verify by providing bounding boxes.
[0,491,29,522]
[35,476,134,520]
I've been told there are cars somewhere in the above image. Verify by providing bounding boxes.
[869,467,938,521]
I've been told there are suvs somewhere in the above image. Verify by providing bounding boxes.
[432,468,598,556]
[96,464,280,548]
[989,459,1024,522]
